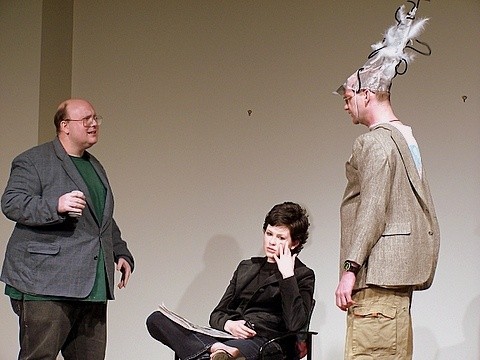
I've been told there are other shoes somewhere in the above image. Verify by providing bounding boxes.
[210,349,245,360]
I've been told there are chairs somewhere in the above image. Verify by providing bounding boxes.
[257,299,318,360]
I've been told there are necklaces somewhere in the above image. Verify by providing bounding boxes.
[389,118,400,123]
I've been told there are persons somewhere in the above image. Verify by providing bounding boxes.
[332,67,440,359]
[0,97,135,360]
[146,202,316,360]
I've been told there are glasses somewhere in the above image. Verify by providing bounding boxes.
[65,115,103,127]
[343,89,375,104]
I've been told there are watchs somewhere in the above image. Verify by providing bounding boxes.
[342,261,358,273]
